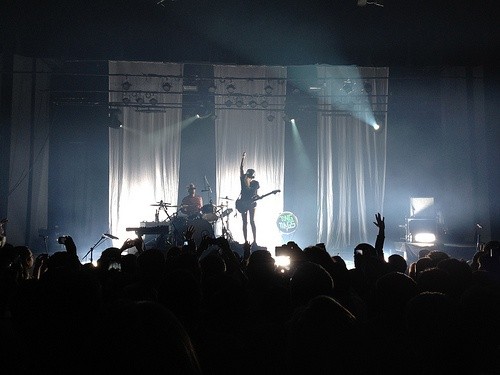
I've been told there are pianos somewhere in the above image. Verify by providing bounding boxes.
[126,227,171,252]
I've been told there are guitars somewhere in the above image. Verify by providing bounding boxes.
[236,190,281,214]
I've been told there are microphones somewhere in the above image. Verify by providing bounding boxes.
[200,190,210,192]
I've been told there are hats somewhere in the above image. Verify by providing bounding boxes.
[188,184,195,192]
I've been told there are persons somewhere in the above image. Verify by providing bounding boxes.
[240,152,260,245]
[179,183,201,216]
[0,213,500,375]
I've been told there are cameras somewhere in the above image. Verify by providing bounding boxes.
[58,237,66,244]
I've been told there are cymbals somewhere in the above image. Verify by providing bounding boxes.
[219,197,233,200]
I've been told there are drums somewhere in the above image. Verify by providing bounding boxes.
[170,215,213,247]
[201,204,219,223]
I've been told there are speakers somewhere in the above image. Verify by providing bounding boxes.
[408,219,435,243]
